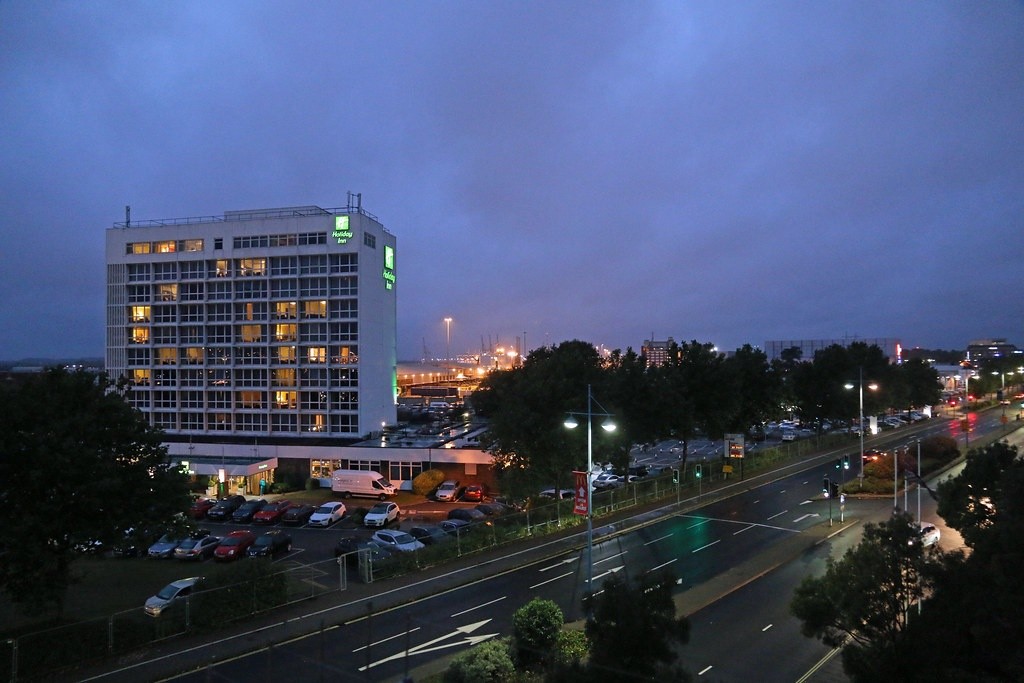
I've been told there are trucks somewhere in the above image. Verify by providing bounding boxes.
[331,469,400,501]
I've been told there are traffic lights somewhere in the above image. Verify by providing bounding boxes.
[823,479,831,499]
[843,454,850,471]
[696,465,702,478]
[832,481,839,497]
[672,469,680,484]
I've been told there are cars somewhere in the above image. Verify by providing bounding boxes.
[592,462,674,490]
[336,502,513,573]
[907,521,941,547]
[187,494,347,528]
[538,489,576,502]
[434,478,490,503]
[751,410,929,440]
[80,527,293,563]
[144,576,207,618]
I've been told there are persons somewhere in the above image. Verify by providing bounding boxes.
[823,474,838,489]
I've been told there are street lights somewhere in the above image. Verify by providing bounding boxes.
[443,317,453,396]
[842,368,881,475]
[990,370,1015,430]
[952,374,981,448]
[563,383,617,600]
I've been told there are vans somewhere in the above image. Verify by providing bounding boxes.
[430,402,450,408]
